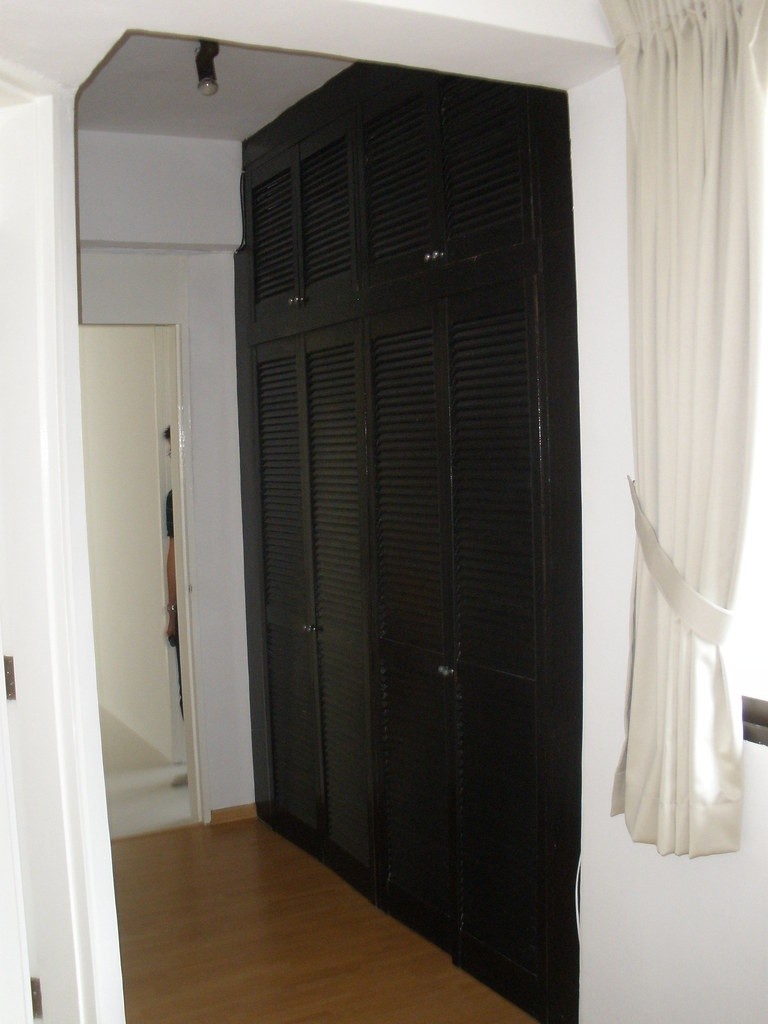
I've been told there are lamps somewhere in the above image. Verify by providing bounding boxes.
[194,40,220,96]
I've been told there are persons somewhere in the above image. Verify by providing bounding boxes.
[163,426,188,787]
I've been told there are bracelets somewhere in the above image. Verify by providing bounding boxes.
[167,604,174,611]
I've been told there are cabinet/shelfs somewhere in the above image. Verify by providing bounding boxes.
[234,61,578,1024]
